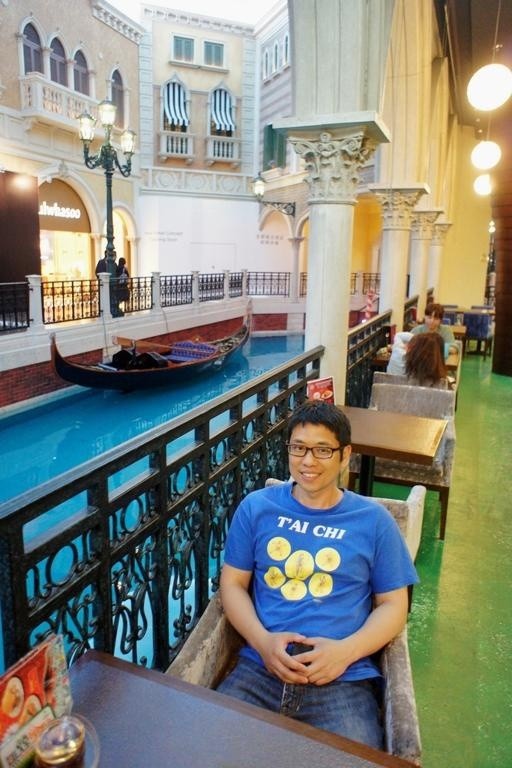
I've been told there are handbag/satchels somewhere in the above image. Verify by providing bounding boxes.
[118,268,128,284]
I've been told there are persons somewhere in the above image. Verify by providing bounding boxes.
[95,250,117,317]
[386,330,446,382]
[410,303,462,356]
[117,258,130,313]
[215,401,421,751]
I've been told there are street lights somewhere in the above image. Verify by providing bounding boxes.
[76,98,136,317]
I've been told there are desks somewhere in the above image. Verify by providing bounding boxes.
[409,323,468,339]
[334,403,448,497]
[372,350,460,413]
[16,649,414,768]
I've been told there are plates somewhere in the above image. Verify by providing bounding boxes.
[70,712,99,768]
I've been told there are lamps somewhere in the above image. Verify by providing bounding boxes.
[467,43,512,113]
[470,115,503,171]
[252,170,294,217]
[473,174,493,195]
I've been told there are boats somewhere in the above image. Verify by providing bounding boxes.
[49,297,253,389]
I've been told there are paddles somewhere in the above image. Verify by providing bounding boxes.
[117,337,213,355]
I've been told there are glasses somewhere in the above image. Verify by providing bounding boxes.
[285,443,342,460]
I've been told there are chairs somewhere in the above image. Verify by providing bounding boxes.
[343,384,456,541]
[462,311,493,362]
[162,578,424,767]
[372,371,449,393]
[258,476,428,619]
